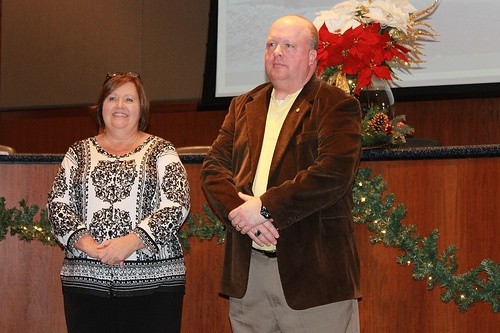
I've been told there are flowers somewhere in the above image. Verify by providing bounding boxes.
[361,103,419,147]
[313,0,440,97]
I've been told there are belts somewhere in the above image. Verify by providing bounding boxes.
[251,245,277,258]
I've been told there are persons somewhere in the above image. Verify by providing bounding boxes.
[46,71,191,333]
[200,14,363,333]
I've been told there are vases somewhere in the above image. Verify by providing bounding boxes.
[350,75,393,150]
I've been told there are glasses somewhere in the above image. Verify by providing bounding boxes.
[103,72,143,86]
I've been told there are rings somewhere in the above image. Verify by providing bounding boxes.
[238,224,242,229]
[254,230,261,237]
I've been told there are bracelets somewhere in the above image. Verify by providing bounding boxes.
[260,202,272,219]
